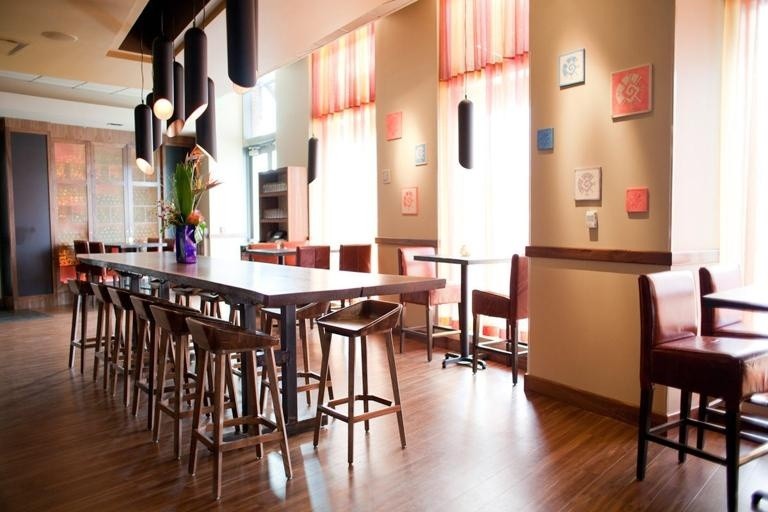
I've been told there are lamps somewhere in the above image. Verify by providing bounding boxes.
[458,1,475,170]
[133,0,259,176]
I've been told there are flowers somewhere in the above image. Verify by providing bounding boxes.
[154,147,222,244]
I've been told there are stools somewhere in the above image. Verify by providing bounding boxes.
[148,304,216,461]
[316,297,406,465]
[129,294,177,420]
[186,314,293,501]
[149,275,338,419]
[107,286,152,407]
[69,278,125,375]
[89,280,128,392]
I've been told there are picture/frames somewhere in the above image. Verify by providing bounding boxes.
[625,188,649,213]
[537,127,554,150]
[384,111,403,141]
[414,143,428,166]
[558,48,585,87]
[609,63,653,119]
[573,166,601,201]
[401,187,419,215]
[382,168,390,184]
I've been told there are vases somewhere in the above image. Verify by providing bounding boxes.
[176,223,198,264]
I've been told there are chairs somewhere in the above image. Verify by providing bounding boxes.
[697,266,768,450]
[74,238,177,307]
[637,268,768,512]
[472,253,529,383]
[249,241,371,313]
[397,246,463,361]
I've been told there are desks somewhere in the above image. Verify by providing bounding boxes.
[702,281,768,313]
[415,254,511,370]
[77,250,446,443]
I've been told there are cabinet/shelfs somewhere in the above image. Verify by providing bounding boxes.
[258,166,308,243]
